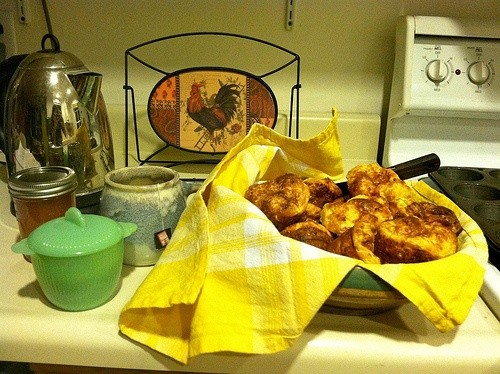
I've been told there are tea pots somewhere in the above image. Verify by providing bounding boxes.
[11,208,139,313]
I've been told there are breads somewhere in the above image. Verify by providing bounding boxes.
[243,162,464,263]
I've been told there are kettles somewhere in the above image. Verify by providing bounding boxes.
[0,34,115,215]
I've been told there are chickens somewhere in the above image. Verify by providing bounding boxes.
[186,79,241,146]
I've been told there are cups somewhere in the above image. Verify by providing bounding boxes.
[7,164,79,264]
[98,165,205,268]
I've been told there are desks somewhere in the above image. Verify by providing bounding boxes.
[0,190,500,373]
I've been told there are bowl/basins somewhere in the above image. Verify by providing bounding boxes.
[313,263,415,316]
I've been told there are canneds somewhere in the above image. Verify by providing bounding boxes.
[7,165,79,264]
